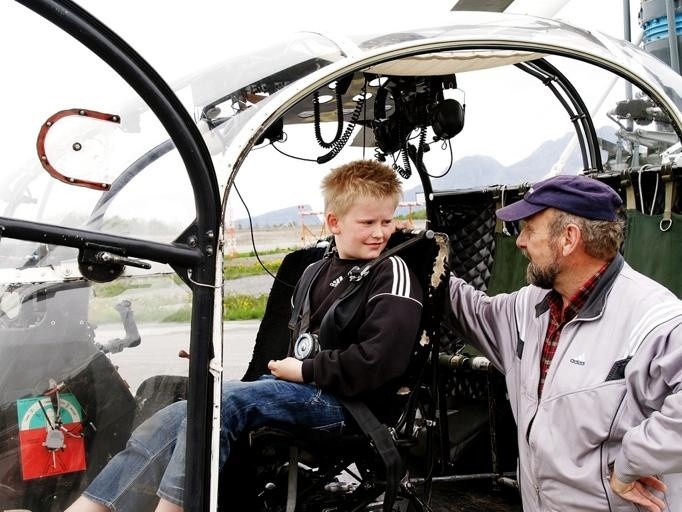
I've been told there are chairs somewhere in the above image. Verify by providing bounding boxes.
[124,225,450,510]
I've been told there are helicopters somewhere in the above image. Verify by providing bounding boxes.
[0,0,682,511]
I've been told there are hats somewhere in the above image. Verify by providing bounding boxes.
[495,172,630,226]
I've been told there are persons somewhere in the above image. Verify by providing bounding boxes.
[389,174,682,512]
[60,159,424,512]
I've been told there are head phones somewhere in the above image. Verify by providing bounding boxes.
[372,74,466,155]
